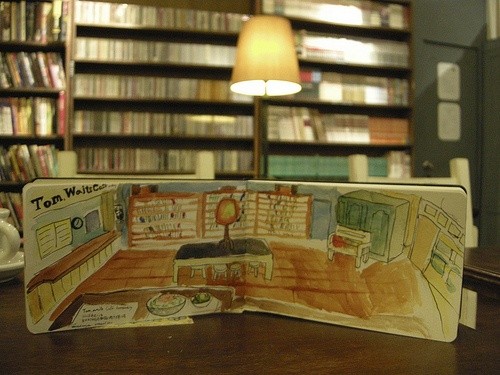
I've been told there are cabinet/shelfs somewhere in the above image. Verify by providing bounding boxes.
[0,0,418,249]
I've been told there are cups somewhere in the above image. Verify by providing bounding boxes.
[0,208,21,264]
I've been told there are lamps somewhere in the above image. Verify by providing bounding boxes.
[228,14,302,179]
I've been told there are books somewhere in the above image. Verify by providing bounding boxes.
[267,105,410,146]
[75,36,237,67]
[74,73,254,103]
[0,144,59,182]
[0,90,65,136]
[262,0,408,31]
[0,51,66,89]
[73,0,245,33]
[0,192,23,238]
[0,0,69,44]
[288,68,409,107]
[292,30,410,69]
[74,110,254,139]
[266,150,412,177]
[22,176,468,343]
[74,147,254,173]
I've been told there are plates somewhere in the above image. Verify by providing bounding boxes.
[0,250,26,283]
